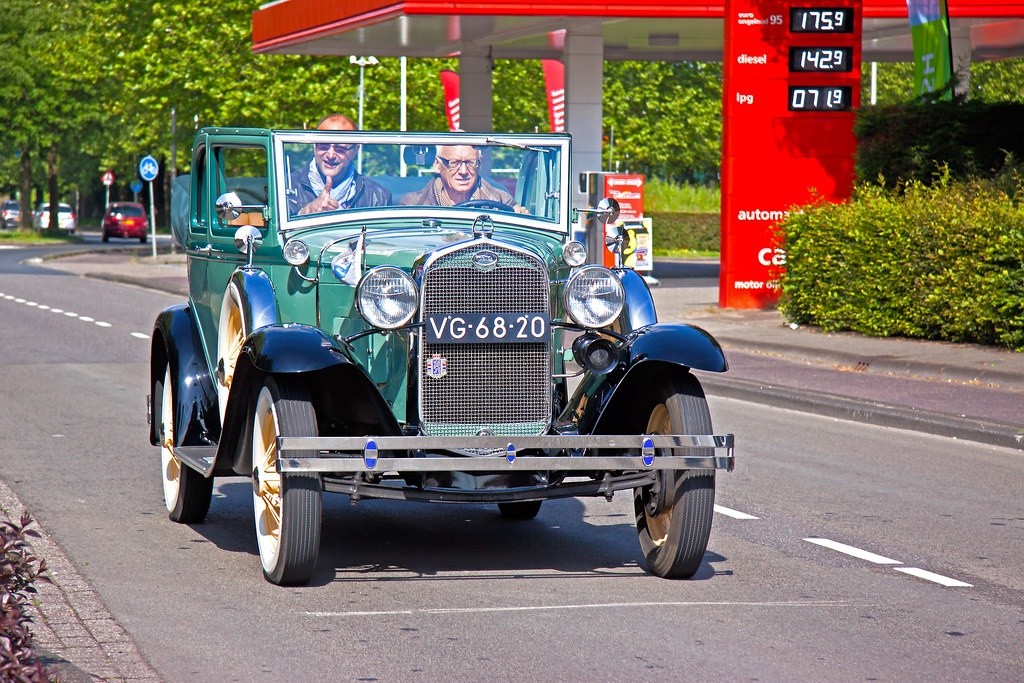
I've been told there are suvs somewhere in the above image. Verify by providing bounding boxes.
[0,201,22,229]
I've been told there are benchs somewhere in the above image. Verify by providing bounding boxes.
[170,175,510,250]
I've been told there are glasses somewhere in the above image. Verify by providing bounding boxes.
[316,143,356,154]
[438,156,481,169]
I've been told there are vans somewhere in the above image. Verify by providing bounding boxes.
[32,202,76,235]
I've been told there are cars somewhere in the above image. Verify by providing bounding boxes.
[147,125,734,585]
[102,202,149,243]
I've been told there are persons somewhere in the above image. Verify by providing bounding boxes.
[284,113,392,215]
[397,144,531,217]
